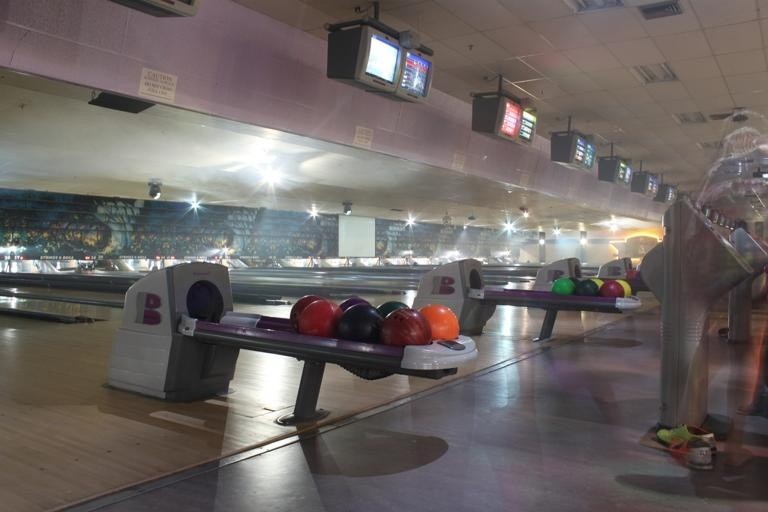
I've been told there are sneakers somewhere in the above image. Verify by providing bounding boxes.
[670,436,716,470]
[656,423,718,455]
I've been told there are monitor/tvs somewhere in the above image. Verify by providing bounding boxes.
[624,165,631,183]
[327,26,403,93]
[656,184,679,201]
[396,48,434,103]
[551,134,587,165]
[472,97,522,141]
[517,109,537,146]
[598,159,627,183]
[583,142,597,169]
[631,172,658,193]
